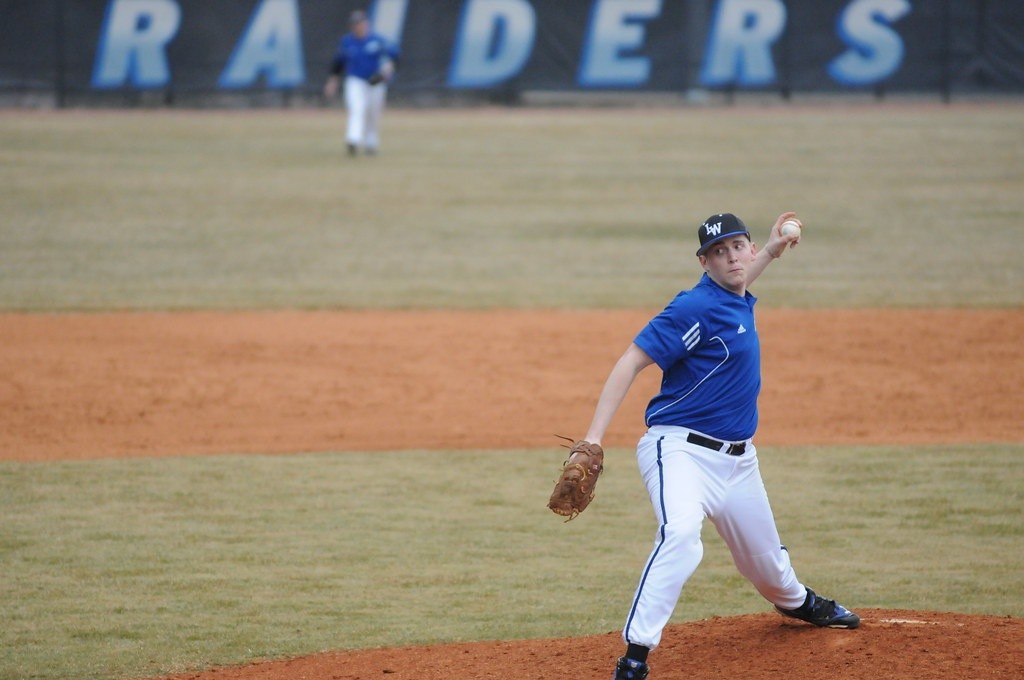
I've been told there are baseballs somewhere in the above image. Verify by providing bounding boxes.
[779,220,801,241]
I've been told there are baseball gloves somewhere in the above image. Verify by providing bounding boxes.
[547,439,604,519]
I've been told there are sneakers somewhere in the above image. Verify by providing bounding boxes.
[614,657,649,680]
[772,583,859,628]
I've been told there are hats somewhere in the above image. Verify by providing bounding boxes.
[695,213,751,259]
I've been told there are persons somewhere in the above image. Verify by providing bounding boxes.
[547,212,861,680]
[325,9,399,156]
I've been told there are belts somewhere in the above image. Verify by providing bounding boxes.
[686,432,746,456]
